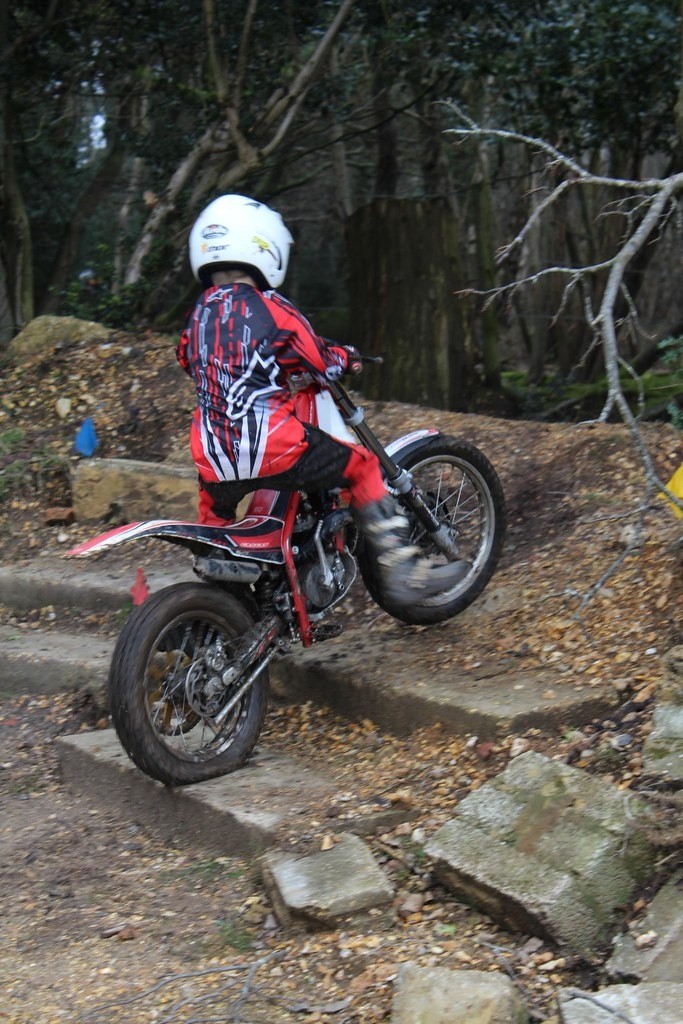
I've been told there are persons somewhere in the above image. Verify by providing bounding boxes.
[174,193,475,609]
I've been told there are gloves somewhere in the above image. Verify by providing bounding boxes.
[341,344,362,375]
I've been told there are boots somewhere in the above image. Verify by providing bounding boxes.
[355,495,474,605]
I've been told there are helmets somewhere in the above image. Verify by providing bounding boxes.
[188,194,296,289]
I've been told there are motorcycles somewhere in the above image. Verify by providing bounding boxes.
[59,339,508,785]
